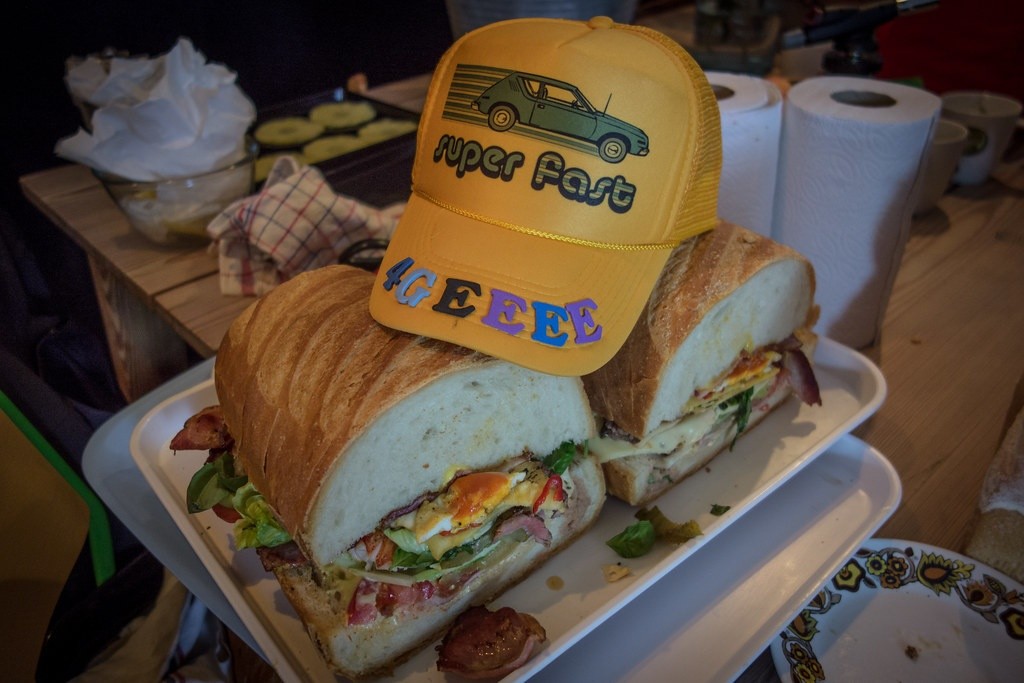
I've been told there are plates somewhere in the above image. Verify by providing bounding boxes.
[771,538,1024,683]
[81,336,902,683]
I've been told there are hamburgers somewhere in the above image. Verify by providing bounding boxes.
[165,262,609,683]
[583,222,827,514]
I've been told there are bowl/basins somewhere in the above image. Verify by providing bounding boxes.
[92,136,260,249]
[915,119,969,211]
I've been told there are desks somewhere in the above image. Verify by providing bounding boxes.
[22,7,1023,683]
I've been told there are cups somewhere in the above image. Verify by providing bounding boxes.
[940,92,1021,186]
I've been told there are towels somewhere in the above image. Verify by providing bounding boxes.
[204,154,396,314]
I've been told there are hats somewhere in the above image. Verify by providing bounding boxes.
[370,16,719,378]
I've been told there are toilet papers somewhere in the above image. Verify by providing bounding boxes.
[702,74,946,350]
